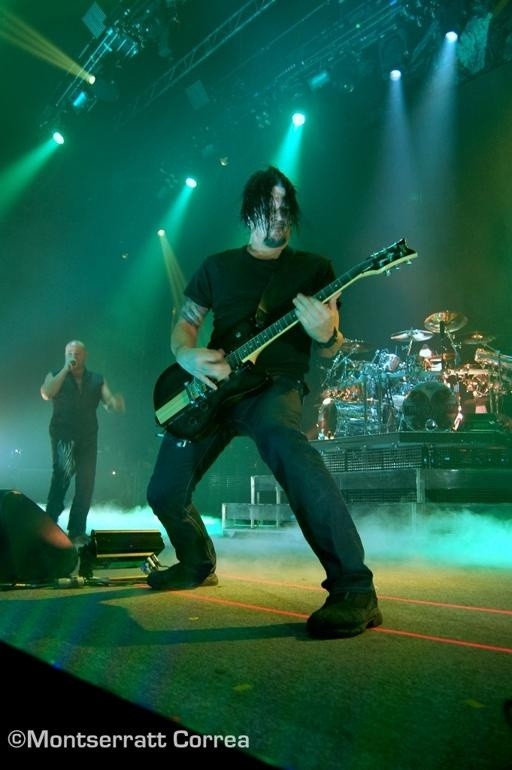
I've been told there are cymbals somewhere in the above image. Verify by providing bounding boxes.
[455,331,496,346]
[424,312,468,333]
[340,338,374,353]
[389,330,434,341]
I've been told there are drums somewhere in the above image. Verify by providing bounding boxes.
[402,383,460,432]
[383,354,401,372]
[318,397,381,438]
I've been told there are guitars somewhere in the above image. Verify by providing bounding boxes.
[153,238,418,449]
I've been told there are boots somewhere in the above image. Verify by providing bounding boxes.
[307,586,383,638]
[146,561,219,589]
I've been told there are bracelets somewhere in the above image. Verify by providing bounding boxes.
[320,326,338,349]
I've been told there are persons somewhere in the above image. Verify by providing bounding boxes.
[40,340,123,549]
[146,166,383,639]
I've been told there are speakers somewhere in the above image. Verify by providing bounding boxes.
[78,529,165,569]
[0,489,78,584]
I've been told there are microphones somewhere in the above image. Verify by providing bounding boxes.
[68,360,76,369]
